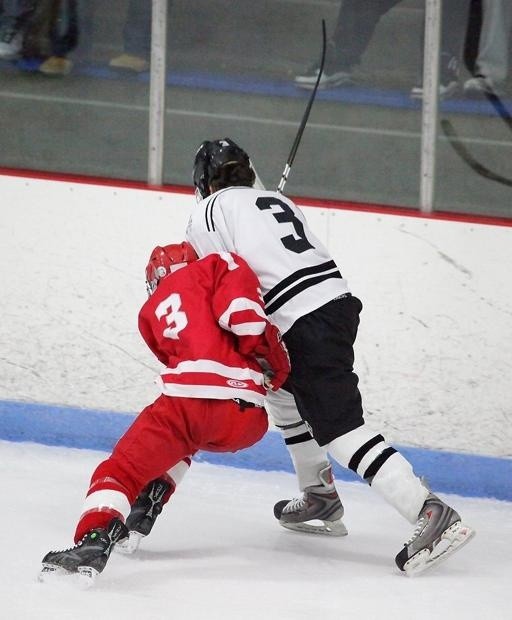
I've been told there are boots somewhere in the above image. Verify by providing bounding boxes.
[43,518,128,575]
[124,475,171,538]
[274,463,344,525]
[395,495,462,572]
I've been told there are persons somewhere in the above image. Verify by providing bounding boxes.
[40,0,153,76]
[463,0,512,101]
[181,140,460,571]
[294,1,483,101]
[0,0,51,60]
[42,240,289,578]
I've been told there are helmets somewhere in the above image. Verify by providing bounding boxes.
[145,242,201,298]
[192,136,256,202]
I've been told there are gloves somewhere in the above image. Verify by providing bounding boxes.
[256,323,291,392]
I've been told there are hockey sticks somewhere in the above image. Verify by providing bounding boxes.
[276,19,327,193]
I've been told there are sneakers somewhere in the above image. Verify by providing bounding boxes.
[1,26,27,58]
[39,56,78,75]
[464,74,506,97]
[410,78,462,102]
[294,62,352,91]
[108,53,149,73]
